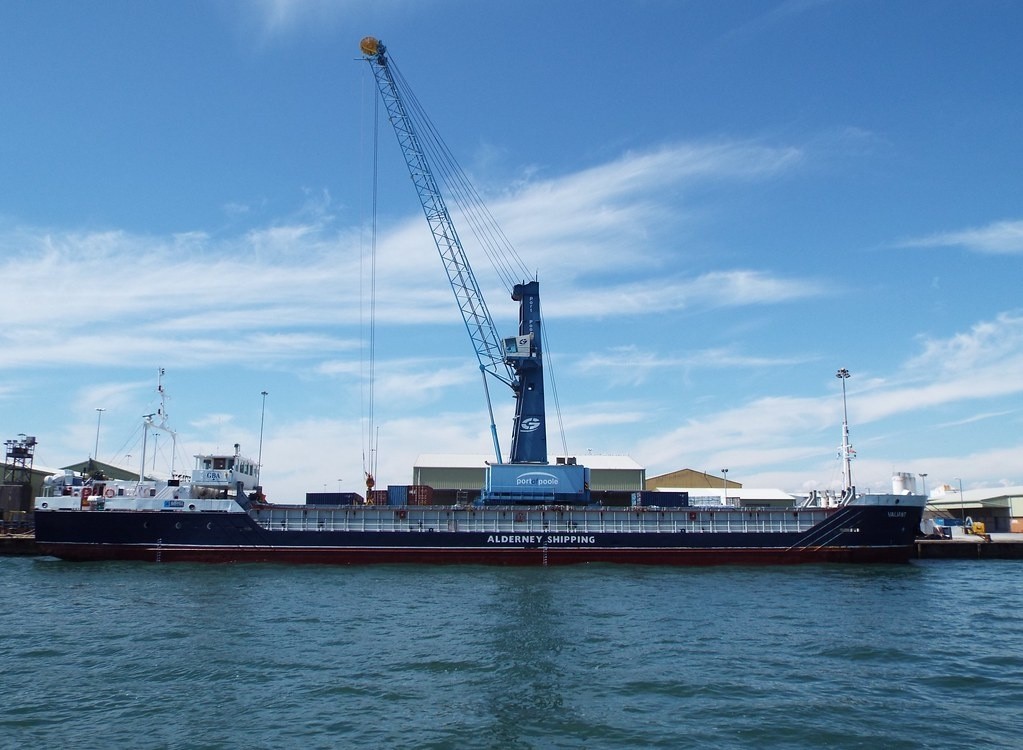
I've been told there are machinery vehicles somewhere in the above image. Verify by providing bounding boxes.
[352,33,595,506]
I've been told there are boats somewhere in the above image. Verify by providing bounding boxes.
[27,420,930,568]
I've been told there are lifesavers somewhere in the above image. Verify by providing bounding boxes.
[429,528,433,532]
[105,488,116,498]
[681,528,685,533]
[82,488,92,496]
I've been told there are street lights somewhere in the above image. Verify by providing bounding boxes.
[918,472,928,495]
[952,476,966,526]
[256,391,271,486]
[92,407,107,481]
[835,367,853,488]
[720,469,730,505]
[153,431,161,480]
[192,455,199,468]
